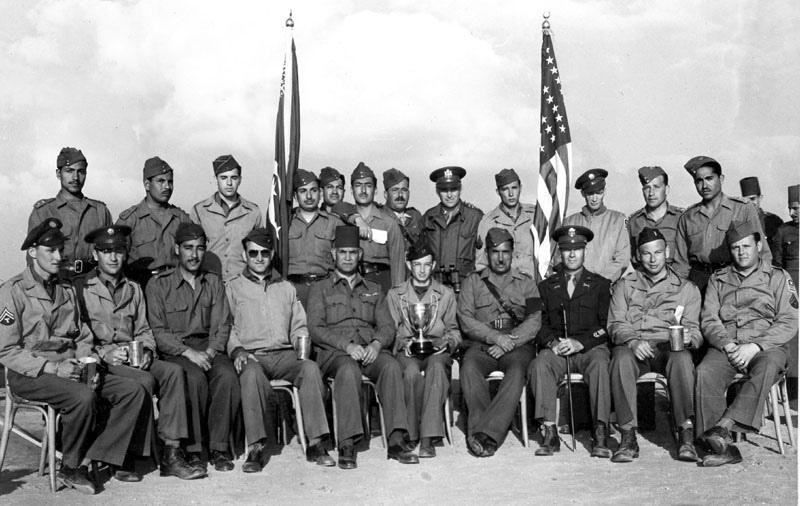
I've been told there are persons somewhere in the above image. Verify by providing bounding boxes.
[1,148,799,494]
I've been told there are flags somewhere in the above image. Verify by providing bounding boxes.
[265,30,301,282]
[530,30,574,279]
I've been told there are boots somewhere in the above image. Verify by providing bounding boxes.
[534,422,561,457]
[613,427,640,463]
[589,419,612,459]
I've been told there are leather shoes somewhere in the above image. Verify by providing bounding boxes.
[699,432,727,454]
[387,438,439,465]
[677,435,697,461]
[701,445,742,467]
[305,441,336,468]
[158,444,235,482]
[55,464,97,495]
[338,442,358,471]
[465,430,500,458]
[241,442,267,474]
[109,462,144,484]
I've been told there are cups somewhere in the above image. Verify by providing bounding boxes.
[77,356,97,385]
[669,325,685,351]
[122,340,144,368]
[296,336,311,361]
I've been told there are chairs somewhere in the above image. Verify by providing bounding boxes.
[2,343,798,494]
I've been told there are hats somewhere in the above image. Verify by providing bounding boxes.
[333,224,362,250]
[428,165,467,189]
[55,145,88,169]
[637,226,667,248]
[493,168,522,188]
[290,160,412,192]
[683,153,720,177]
[243,226,274,252]
[23,216,69,250]
[738,175,762,199]
[637,164,670,187]
[484,226,514,251]
[405,241,436,260]
[141,155,174,181]
[787,184,800,205]
[84,224,133,252]
[173,223,209,244]
[550,225,596,250]
[725,217,759,245]
[574,167,610,195]
[211,154,241,177]
